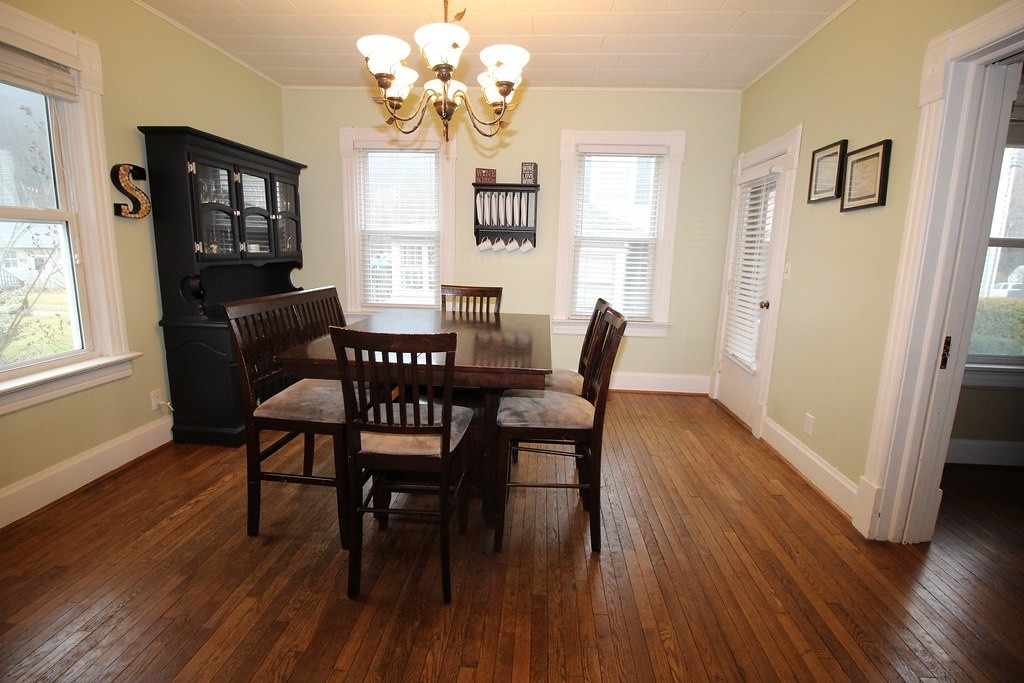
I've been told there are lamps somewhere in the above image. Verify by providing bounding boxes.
[355,0,531,142]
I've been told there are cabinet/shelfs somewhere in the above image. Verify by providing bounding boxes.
[137,125,305,449]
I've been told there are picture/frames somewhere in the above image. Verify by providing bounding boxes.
[806,140,848,203]
[839,139,893,212]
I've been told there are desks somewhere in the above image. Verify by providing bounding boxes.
[272,309,553,526]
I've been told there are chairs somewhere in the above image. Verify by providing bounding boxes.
[220,284,627,605]
